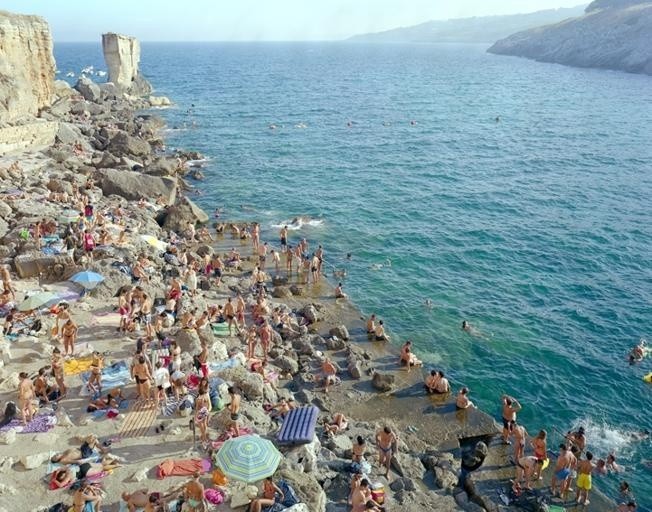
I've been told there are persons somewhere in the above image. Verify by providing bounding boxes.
[0,206,651,512]
[0,138,204,222]
